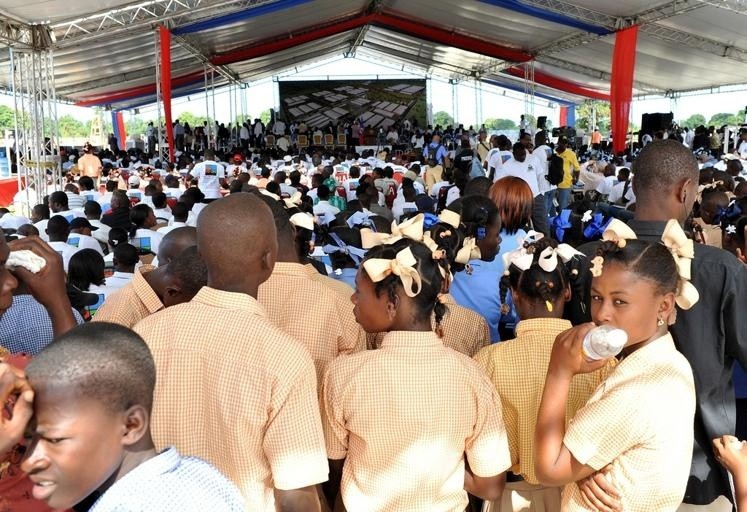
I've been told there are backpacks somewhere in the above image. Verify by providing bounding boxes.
[544,154,565,186]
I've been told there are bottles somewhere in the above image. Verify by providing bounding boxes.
[582,323,629,364]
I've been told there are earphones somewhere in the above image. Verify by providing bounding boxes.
[683,190,687,201]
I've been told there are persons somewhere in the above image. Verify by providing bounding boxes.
[532,217,701,512]
[0,319,256,511]
[318,236,511,512]
[568,139,747,512]
[0,109,745,349]
[0,227,83,511]
[466,238,621,512]
[127,192,331,511]
[710,432,747,512]
[254,192,386,511]
